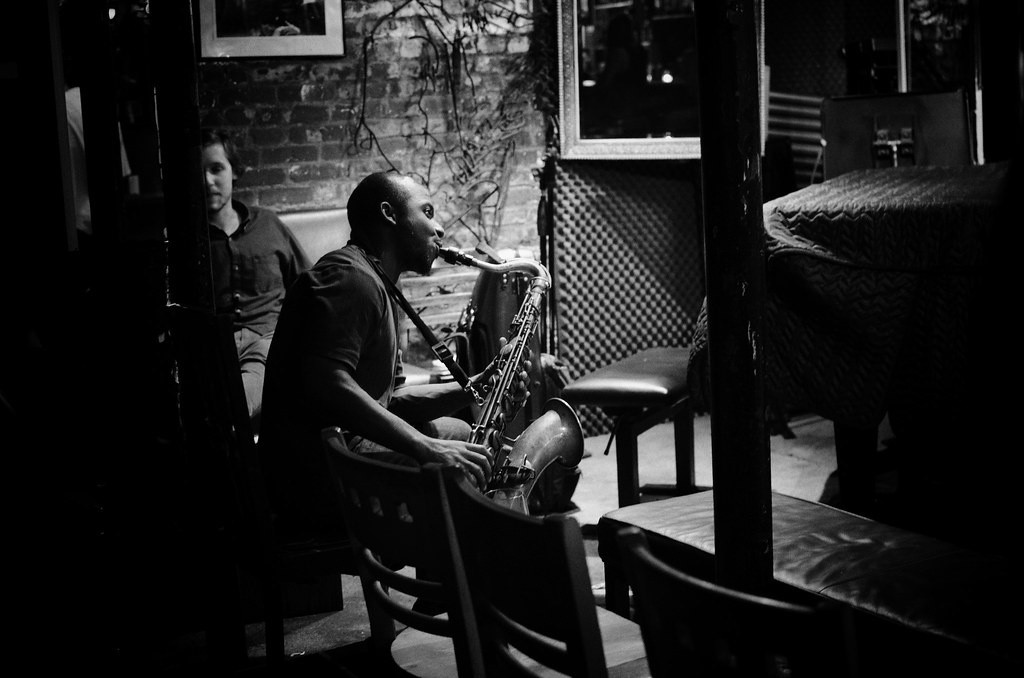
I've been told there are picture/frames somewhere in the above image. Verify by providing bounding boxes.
[199,0,347,60]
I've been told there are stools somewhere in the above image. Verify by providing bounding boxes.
[563,345,709,506]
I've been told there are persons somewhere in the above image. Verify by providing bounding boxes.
[199,130,312,419]
[256,168,534,609]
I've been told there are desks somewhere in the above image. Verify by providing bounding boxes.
[598,488,1024,678]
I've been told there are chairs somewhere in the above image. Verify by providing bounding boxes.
[321,425,841,678]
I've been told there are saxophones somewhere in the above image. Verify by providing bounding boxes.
[429,241,585,517]
